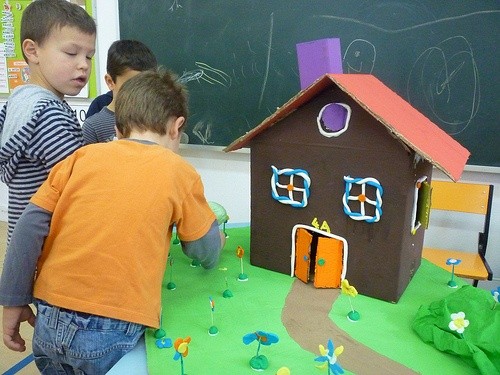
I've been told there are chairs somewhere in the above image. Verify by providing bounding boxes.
[419,178,494,287]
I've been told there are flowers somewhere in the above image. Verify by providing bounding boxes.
[449,312,471,335]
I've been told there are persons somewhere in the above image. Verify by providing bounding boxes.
[0,1,98,281]
[81,39,157,147]
[0,66,227,375]
[84,88,114,123]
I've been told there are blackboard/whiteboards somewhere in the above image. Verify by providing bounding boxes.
[116,0,500,175]
